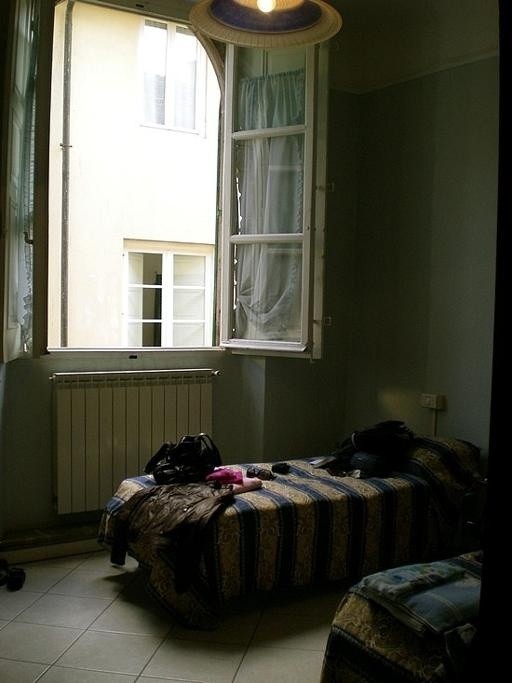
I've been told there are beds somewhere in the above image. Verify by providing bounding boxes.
[319,549,487,681]
[103,436,487,614]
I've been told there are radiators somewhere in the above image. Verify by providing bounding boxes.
[47,368,214,513]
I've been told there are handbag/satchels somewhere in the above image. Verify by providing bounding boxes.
[145,433,225,484]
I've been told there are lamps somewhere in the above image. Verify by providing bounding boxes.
[188,0,342,49]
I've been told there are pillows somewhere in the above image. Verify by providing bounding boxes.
[412,437,481,476]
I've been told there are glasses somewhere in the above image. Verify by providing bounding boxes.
[247,468,277,480]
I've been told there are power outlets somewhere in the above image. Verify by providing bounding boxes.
[421,393,445,410]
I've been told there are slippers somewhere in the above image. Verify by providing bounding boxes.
[7,568,25,590]
[0,558,9,586]
